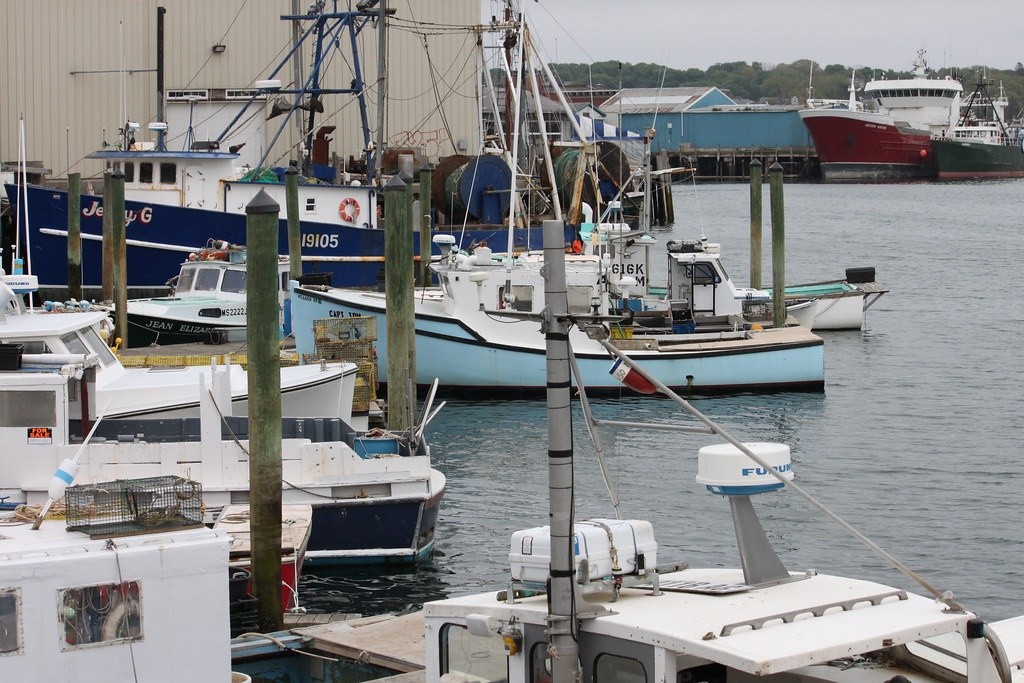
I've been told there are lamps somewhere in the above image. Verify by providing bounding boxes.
[212,44,226,52]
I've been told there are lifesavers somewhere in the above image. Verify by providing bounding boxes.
[340,198,360,222]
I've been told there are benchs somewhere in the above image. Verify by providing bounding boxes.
[671,308,695,325]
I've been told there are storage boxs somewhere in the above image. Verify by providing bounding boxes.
[609,307,635,324]
[295,270,333,288]
[0,343,24,370]
[609,326,637,340]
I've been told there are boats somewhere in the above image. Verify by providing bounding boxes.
[796,59,967,183]
[420,439,1024,683]
[930,63,1024,180]
[0,2,883,683]
